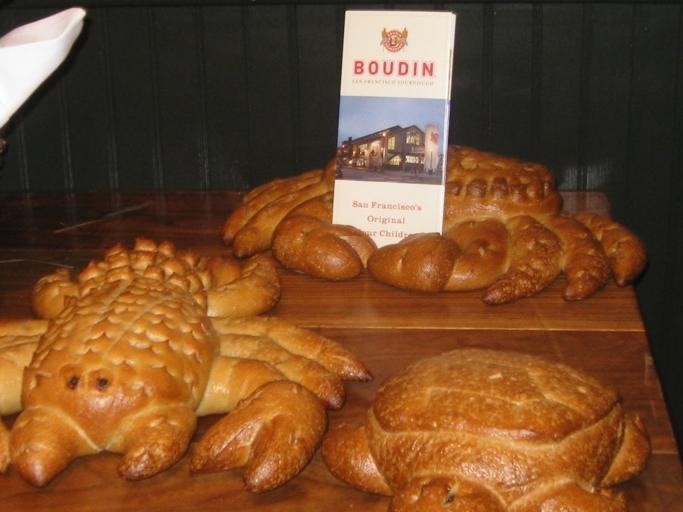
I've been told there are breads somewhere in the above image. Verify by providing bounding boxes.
[0,237,375,494]
[322,346,650,512]
[223,143,646,305]
